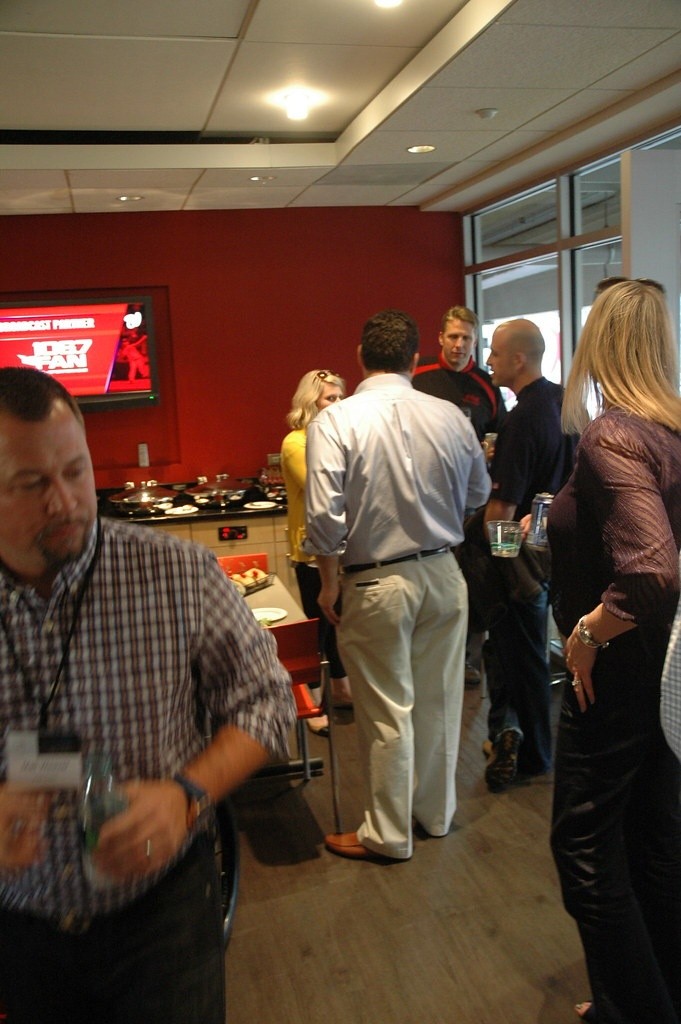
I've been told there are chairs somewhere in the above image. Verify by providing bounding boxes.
[224,617,343,835]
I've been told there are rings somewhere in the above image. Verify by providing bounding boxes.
[572,680,583,686]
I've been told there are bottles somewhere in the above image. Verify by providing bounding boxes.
[75,736,136,888]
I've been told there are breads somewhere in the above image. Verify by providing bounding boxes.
[229,568,267,589]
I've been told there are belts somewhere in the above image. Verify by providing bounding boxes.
[341,544,449,574]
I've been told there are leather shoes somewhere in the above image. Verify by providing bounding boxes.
[324,830,412,863]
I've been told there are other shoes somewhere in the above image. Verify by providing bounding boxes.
[332,701,354,710]
[483,727,523,792]
[306,715,330,737]
[466,665,484,685]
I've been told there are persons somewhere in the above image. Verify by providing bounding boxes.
[412,303,508,690]
[304,310,493,861]
[541,275,681,1023]
[280,369,356,737]
[0,365,299,1024]
[464,319,591,793]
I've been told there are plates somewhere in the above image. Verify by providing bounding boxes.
[164,506,200,514]
[252,607,288,622]
[243,501,278,509]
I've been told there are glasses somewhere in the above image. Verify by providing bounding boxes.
[595,275,667,296]
[312,370,341,383]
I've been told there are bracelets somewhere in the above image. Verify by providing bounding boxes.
[578,615,610,650]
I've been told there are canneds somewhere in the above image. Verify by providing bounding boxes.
[526,492,554,549]
[484,434,498,447]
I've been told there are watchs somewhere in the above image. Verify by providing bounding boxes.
[174,775,211,829]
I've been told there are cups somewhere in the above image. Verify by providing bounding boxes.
[483,432,498,464]
[485,519,525,558]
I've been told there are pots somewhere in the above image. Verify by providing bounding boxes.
[108,478,181,518]
[181,473,254,510]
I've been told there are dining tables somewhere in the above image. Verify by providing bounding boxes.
[234,573,309,628]
